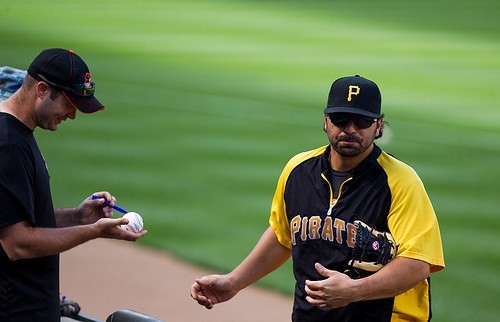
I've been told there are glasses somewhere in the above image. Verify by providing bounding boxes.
[326,113,381,129]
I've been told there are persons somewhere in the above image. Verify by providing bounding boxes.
[190,74,445,322]
[0,49,149,322]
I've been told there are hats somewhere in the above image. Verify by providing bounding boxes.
[28,48,105,114]
[323,75,381,118]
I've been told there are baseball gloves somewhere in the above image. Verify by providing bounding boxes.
[345,219,397,279]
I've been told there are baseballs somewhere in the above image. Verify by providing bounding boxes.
[121,211,144,233]
